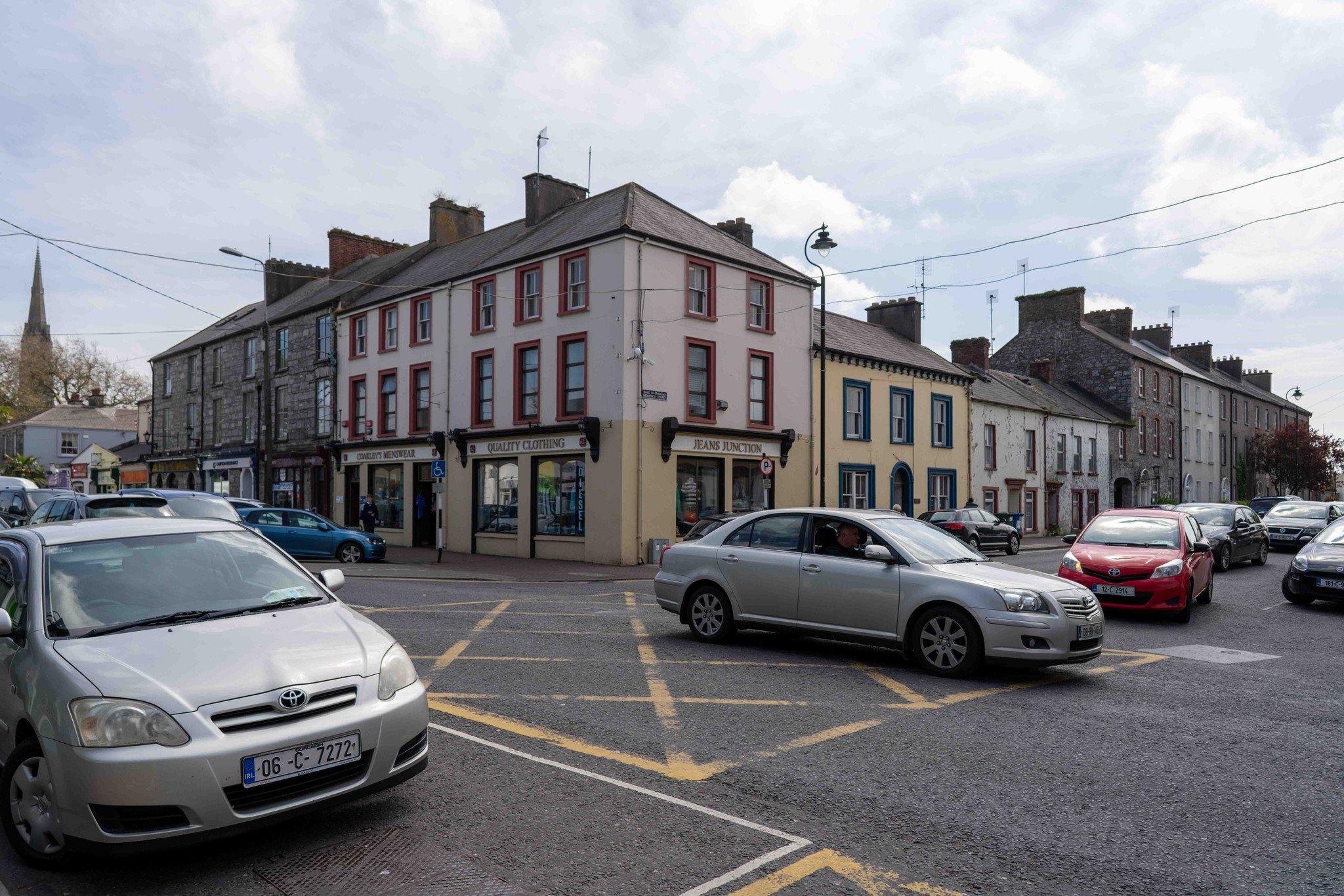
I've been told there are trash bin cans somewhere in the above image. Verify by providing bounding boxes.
[1010,512,1024,539]
[994,512,1015,527]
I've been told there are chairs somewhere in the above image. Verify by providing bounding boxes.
[816,522,868,559]
[290,514,299,527]
[50,547,230,614]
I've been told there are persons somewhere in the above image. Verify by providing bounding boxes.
[817,522,861,561]
[360,493,380,533]
[681,476,701,507]
[892,504,907,517]
[964,498,979,508]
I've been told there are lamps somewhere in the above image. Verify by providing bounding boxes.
[1134,465,1161,494]
[143,430,158,451]
[578,417,585,435]
[627,347,641,363]
[427,432,434,446]
[448,429,456,444]
[185,425,200,446]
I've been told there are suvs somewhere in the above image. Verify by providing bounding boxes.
[220,496,319,530]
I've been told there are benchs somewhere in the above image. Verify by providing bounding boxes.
[736,529,800,549]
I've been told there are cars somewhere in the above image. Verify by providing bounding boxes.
[0,473,247,569]
[237,507,388,563]
[1136,493,1344,572]
[652,508,1108,680]
[1280,516,1344,606]
[1058,507,1216,624]
[915,509,1022,556]
[682,510,784,546]
[0,511,432,873]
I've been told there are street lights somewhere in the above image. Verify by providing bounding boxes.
[802,222,840,507]
[1286,387,1304,497]
[218,245,275,506]
